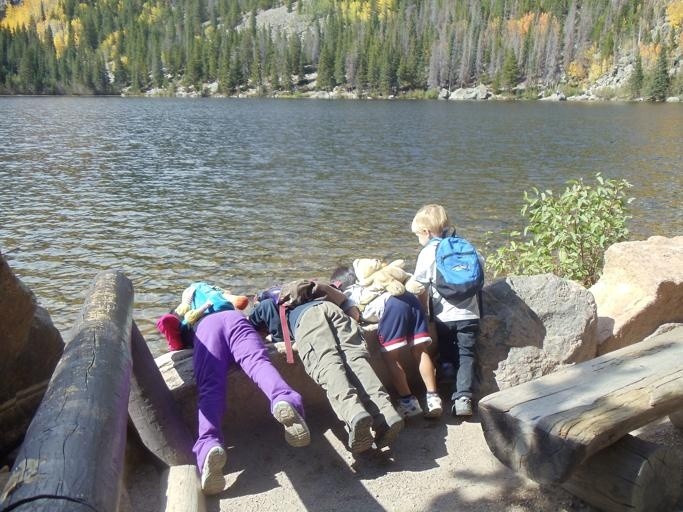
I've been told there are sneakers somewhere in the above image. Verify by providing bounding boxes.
[375,417,405,464]
[423,392,443,419]
[201,446,229,498]
[436,361,459,387]
[453,395,473,417]
[347,410,373,455]
[272,399,312,448]
[394,396,425,421]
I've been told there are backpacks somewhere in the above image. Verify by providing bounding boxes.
[175,281,250,321]
[434,224,485,301]
[353,254,425,307]
[265,278,346,308]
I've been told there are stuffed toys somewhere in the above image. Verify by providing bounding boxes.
[175,282,235,323]
[353,257,426,296]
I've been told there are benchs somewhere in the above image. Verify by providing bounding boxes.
[479,323,683,512]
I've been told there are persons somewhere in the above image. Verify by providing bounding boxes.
[157,282,312,495]
[247,278,406,455]
[330,264,444,420]
[412,202,485,416]
